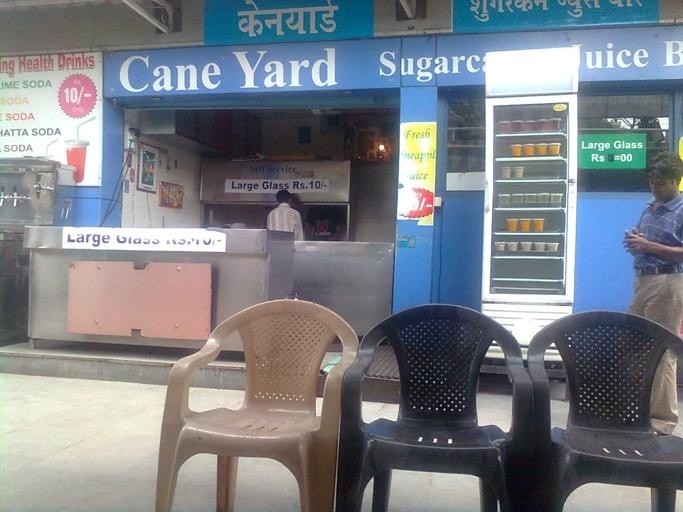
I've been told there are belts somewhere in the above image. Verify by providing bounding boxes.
[634,263,681,276]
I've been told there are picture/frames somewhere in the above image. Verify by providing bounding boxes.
[137,141,161,195]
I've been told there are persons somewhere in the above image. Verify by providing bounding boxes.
[266,190,304,240]
[304,205,345,241]
[623,151,682,436]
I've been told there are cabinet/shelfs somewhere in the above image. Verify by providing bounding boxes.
[216,109,262,159]
[139,109,230,158]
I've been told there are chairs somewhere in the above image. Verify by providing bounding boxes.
[527,310,683,512]
[155,297,361,512]
[334,303,537,512]
